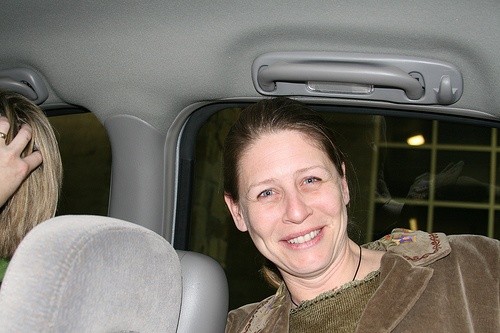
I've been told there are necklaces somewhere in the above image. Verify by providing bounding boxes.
[284,242,362,308]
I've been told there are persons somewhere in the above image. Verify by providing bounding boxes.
[223,96,499,333]
[0,88,61,292]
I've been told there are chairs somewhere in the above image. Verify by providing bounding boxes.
[176,250,229,333]
[0,216,182,333]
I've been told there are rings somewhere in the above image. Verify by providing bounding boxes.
[0,132,6,139]
